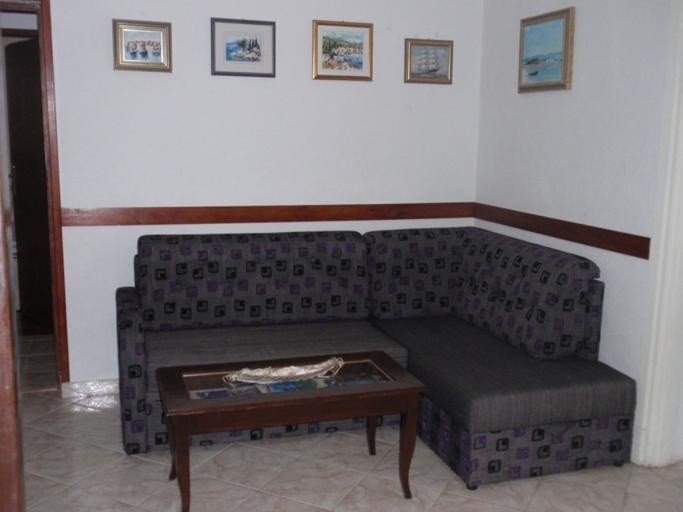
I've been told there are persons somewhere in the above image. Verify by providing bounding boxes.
[126,40,160,61]
[323,40,351,72]
[245,44,261,62]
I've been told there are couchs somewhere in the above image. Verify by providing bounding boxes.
[118,225,639,492]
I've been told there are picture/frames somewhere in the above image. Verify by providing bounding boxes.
[209,17,278,80]
[517,6,576,93]
[403,36,453,85]
[112,18,174,75]
[310,17,375,83]
